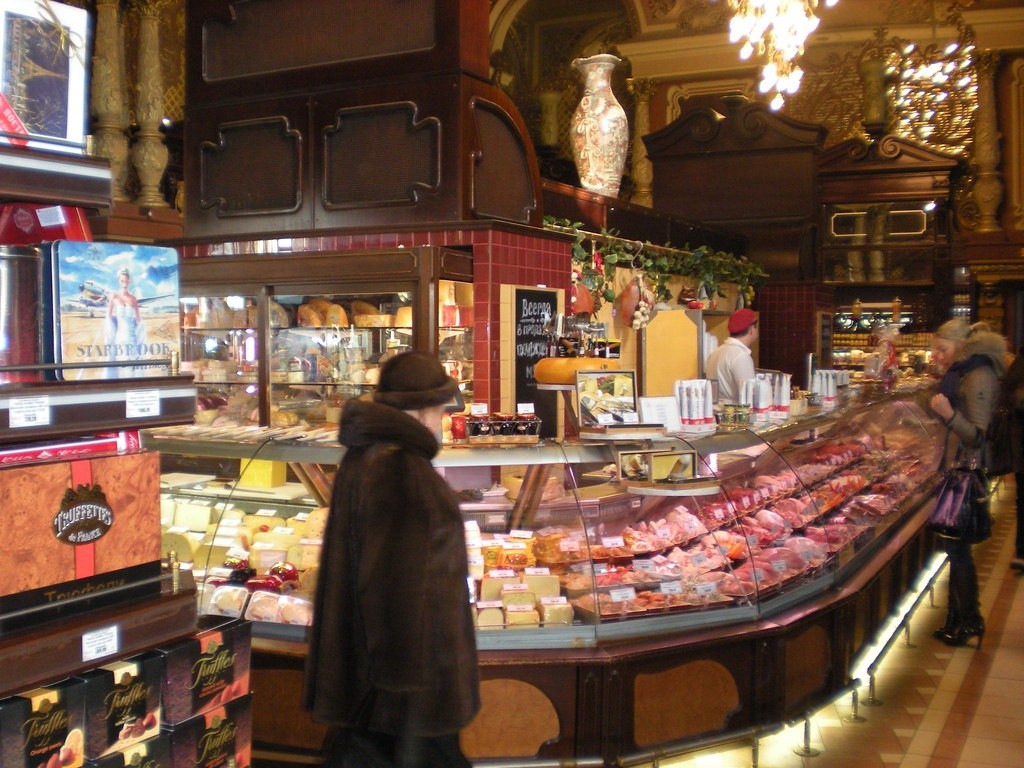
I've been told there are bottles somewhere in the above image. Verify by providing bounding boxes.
[812,332,936,401]
[543,314,608,357]
[952,288,970,323]
[569,53,630,199]
[674,373,791,432]
[467,400,542,436]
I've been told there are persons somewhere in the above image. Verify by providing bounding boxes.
[930,320,1024,646]
[706,308,761,402]
[303,348,487,768]
[105,268,143,377]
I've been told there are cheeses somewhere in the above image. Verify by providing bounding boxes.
[157,498,576,630]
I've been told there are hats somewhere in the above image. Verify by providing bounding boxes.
[728,308,758,333]
[373,351,459,410]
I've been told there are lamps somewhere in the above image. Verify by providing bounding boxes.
[720,0,827,114]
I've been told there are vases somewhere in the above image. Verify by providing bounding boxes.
[569,53,629,200]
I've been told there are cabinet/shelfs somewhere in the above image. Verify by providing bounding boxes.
[178,245,478,431]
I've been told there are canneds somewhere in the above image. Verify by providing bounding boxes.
[722,403,751,431]
[468,412,537,437]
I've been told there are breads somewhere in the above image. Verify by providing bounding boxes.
[182,299,474,427]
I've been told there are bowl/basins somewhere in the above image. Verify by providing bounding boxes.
[688,301,703,309]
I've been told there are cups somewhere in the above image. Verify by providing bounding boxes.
[791,399,808,417]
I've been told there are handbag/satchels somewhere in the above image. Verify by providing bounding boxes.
[927,426,993,544]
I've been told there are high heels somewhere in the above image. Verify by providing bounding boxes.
[932,610,986,649]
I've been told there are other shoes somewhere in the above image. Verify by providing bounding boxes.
[1010,558,1024,576]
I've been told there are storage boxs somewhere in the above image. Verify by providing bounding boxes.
[0,239,252,768]
